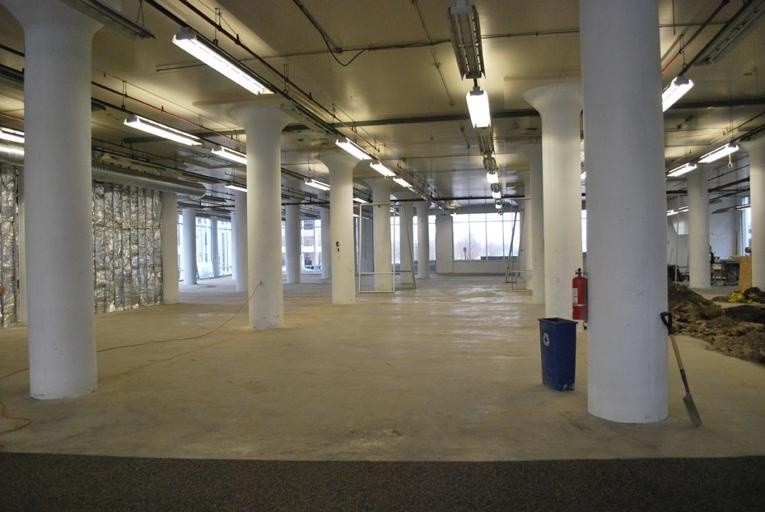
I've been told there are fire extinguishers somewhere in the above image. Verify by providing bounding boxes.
[572,268,587,320]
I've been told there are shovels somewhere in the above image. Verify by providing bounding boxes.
[660,311,702,426]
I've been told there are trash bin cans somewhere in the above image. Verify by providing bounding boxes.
[538,317,578,391]
